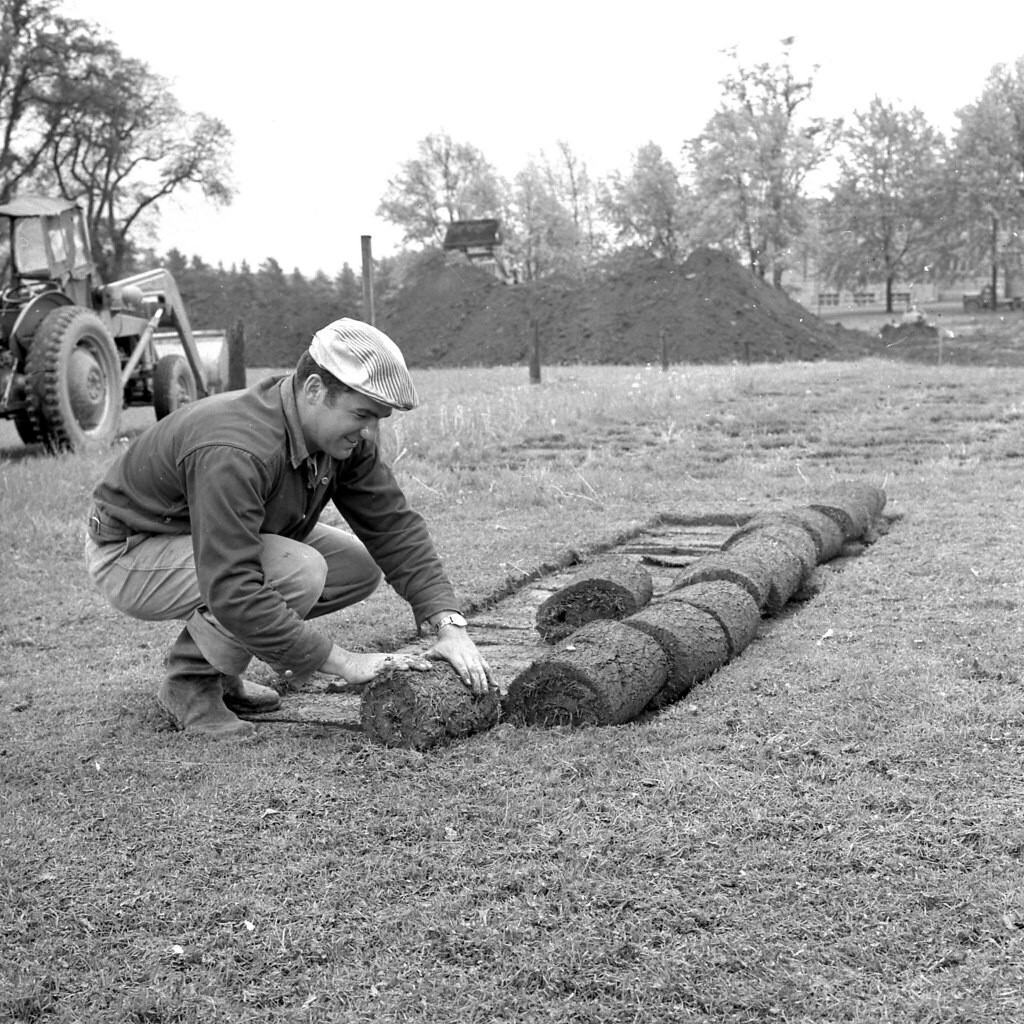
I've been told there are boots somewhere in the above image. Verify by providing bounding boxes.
[157,626,257,744]
[220,672,281,713]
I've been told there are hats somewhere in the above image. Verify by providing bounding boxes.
[309,318,419,411]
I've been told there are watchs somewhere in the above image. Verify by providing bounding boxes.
[435,614,467,633]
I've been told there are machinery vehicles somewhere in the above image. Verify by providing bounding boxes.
[0,198,248,457]
[962,273,1024,314]
[442,216,520,285]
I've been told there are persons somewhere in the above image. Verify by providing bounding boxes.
[84,317,498,740]
